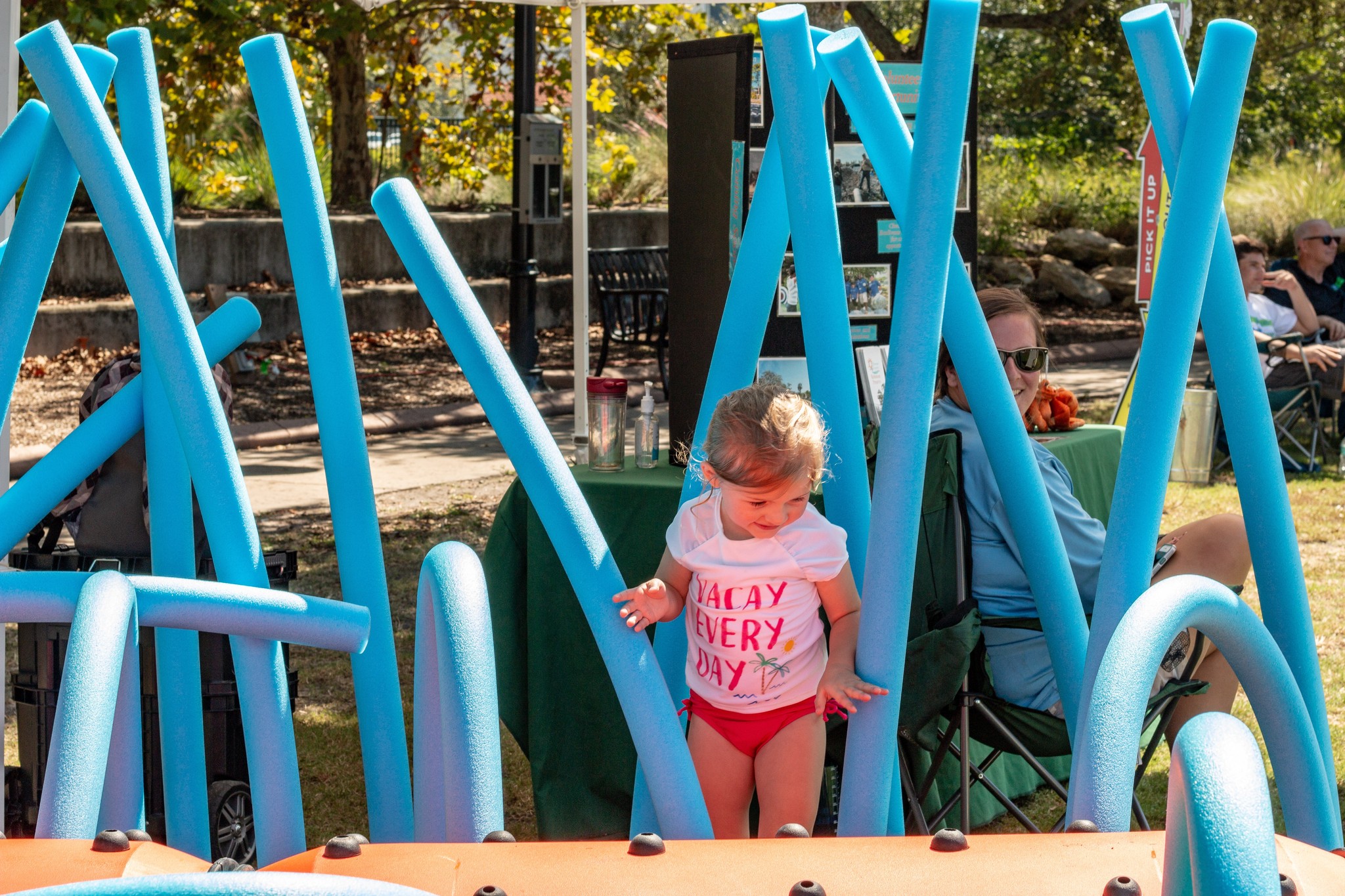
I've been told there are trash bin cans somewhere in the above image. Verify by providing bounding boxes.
[1168,390,1217,483]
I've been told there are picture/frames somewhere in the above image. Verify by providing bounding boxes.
[755,356,812,403]
[750,49,765,128]
[842,263,892,319]
[829,141,890,208]
[776,250,801,317]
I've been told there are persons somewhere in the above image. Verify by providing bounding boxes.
[1232,219,1345,440]
[930,287,1254,752]
[612,378,890,837]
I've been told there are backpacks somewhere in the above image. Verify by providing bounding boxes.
[26,348,233,558]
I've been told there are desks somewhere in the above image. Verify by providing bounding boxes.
[483,426,1122,848]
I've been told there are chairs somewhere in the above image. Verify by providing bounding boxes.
[1206,327,1335,474]
[820,429,1244,835]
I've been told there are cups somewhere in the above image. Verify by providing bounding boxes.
[587,376,628,472]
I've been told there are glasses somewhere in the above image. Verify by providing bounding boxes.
[1298,236,1341,251]
[998,347,1049,373]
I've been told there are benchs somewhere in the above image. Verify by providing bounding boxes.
[587,245,669,403]
